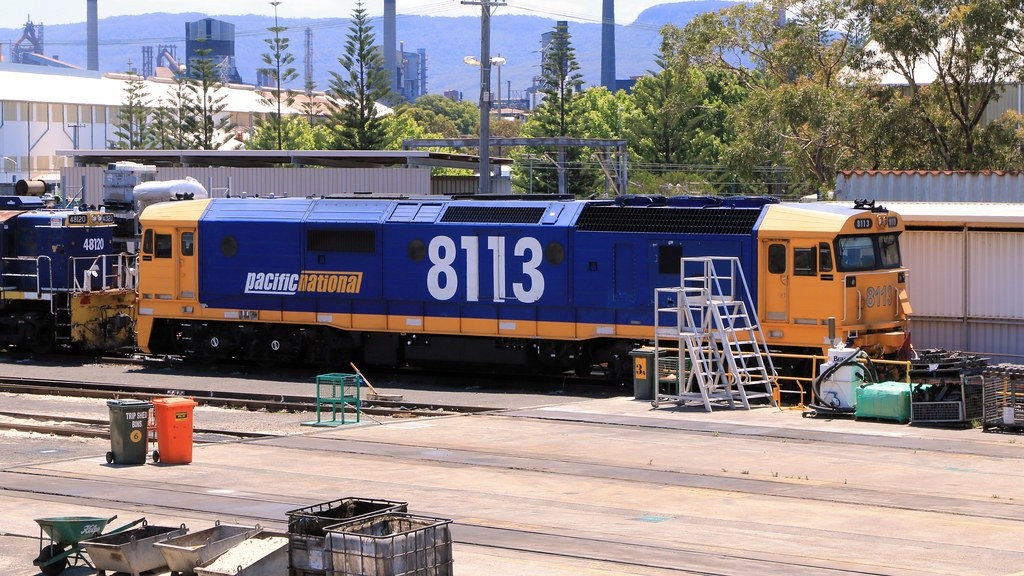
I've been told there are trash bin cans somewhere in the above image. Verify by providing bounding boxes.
[106,398,151,467]
[152,397,198,465]
[628,345,667,402]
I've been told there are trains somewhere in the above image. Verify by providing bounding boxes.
[1,161,921,409]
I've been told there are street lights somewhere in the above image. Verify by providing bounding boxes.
[269,0,282,149]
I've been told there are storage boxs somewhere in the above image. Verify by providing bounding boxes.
[284,497,455,576]
